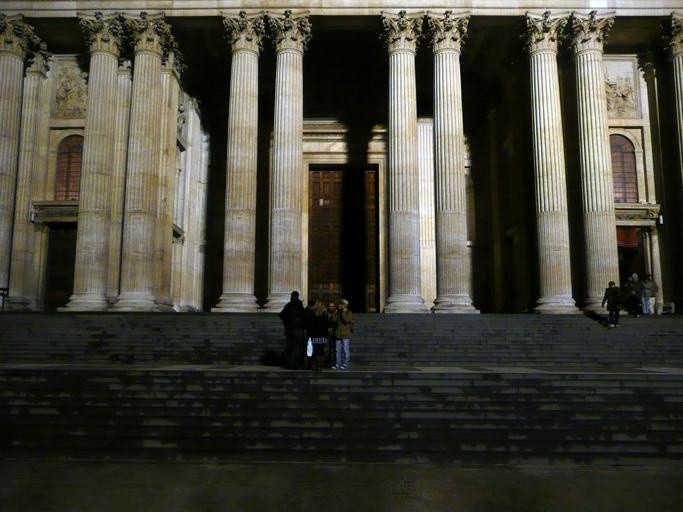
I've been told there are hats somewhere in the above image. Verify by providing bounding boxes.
[339,298,349,305]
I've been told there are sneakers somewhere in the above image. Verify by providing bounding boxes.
[330,363,350,370]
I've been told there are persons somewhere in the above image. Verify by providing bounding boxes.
[602,272,658,328]
[279,290,352,370]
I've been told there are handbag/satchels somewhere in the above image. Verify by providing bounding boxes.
[306,338,314,357]
